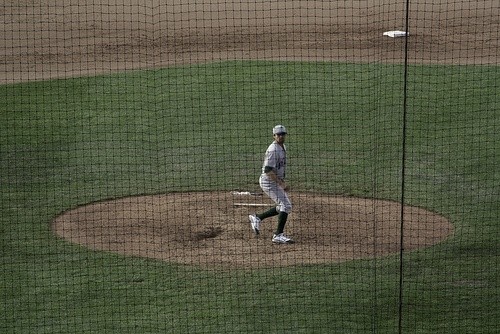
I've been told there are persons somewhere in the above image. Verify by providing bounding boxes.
[248,125,295,243]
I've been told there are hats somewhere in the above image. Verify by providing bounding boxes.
[273,124,287,134]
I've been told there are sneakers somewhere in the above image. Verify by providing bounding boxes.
[272,233,295,243]
[249,214,261,234]
